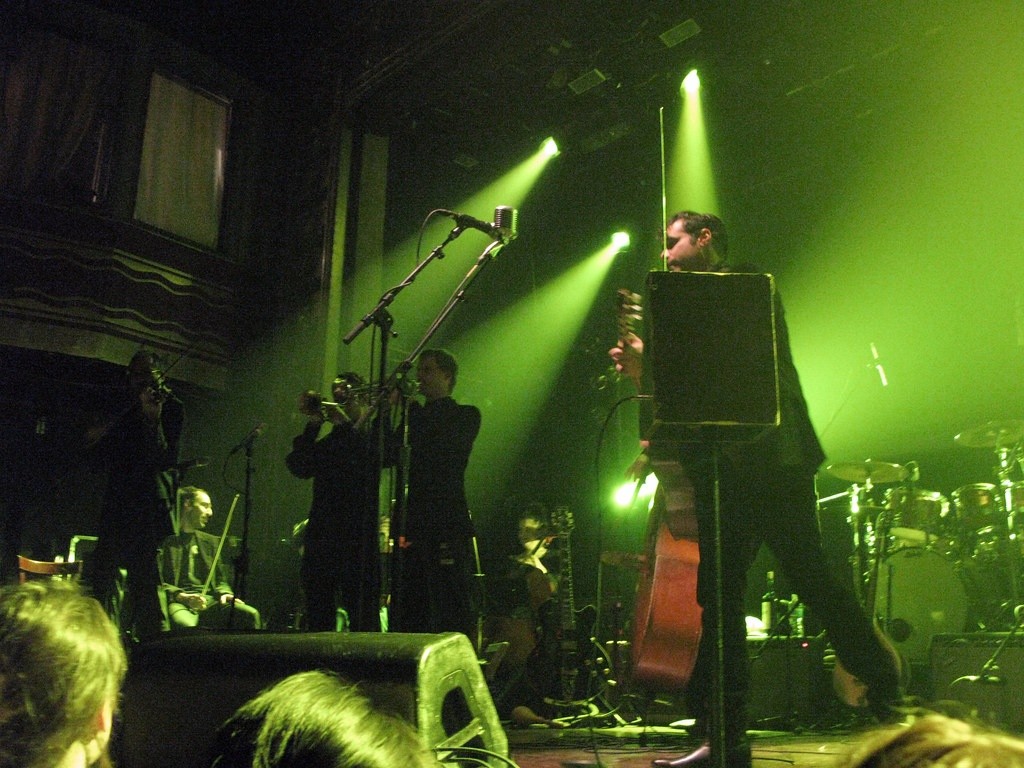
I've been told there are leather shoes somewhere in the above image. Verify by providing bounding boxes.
[867,674,891,720]
[652,733,753,768]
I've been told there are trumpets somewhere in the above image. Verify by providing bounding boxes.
[296,389,352,422]
[331,377,423,408]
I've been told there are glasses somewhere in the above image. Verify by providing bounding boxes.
[127,368,161,378]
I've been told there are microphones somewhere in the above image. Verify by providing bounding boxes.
[494,206,518,237]
[963,675,1005,686]
[177,456,210,470]
[435,209,498,242]
[229,423,269,454]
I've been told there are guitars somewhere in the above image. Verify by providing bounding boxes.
[525,506,614,707]
[832,485,901,708]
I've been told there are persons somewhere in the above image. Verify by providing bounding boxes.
[285,371,382,633]
[608,211,902,768]
[835,713,1024,767]
[0,574,127,768]
[485,501,578,727]
[368,348,481,767]
[193,669,431,768]
[157,486,260,628]
[75,350,185,639]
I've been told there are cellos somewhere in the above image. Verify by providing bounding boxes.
[623,258,704,747]
[469,505,558,711]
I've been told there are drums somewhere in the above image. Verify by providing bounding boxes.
[872,545,994,666]
[1011,480,1024,557]
[878,487,950,545]
[950,482,1004,537]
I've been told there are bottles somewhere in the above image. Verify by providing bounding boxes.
[762,571,778,635]
[51,552,64,582]
[789,594,803,636]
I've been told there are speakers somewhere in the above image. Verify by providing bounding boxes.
[931,631,1024,734]
[118,631,508,768]
[639,269,780,442]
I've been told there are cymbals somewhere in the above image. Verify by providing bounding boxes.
[953,419,1024,448]
[826,459,910,483]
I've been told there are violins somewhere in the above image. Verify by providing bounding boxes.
[135,378,175,404]
